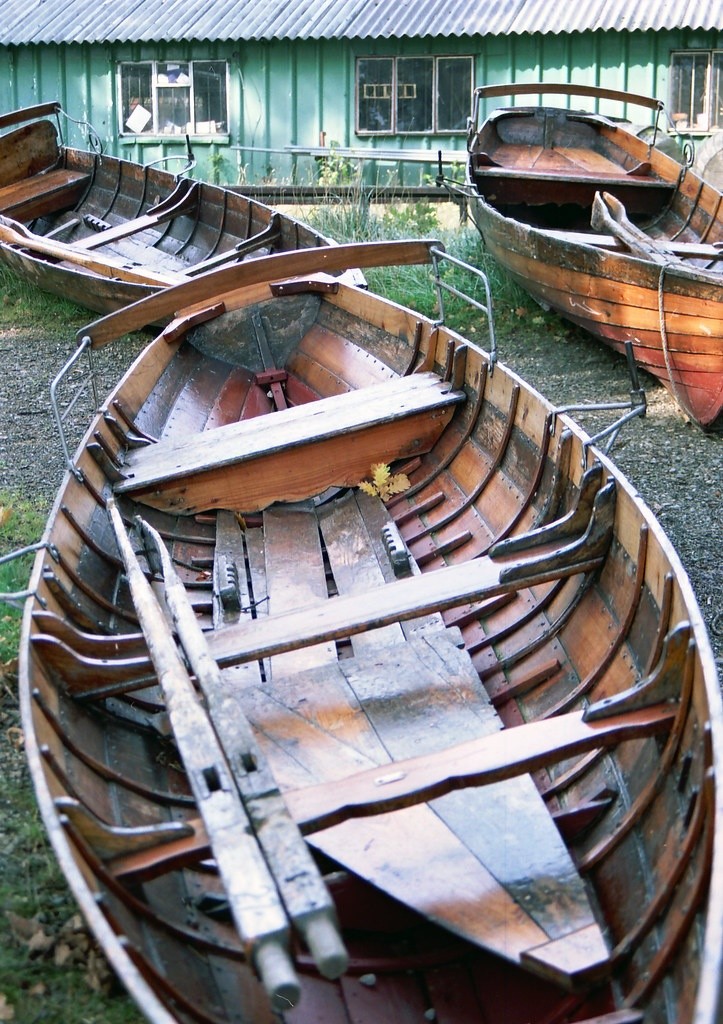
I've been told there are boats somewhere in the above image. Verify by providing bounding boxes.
[0,100,370,331]
[429,83,723,434]
[0,231,723,1024]
